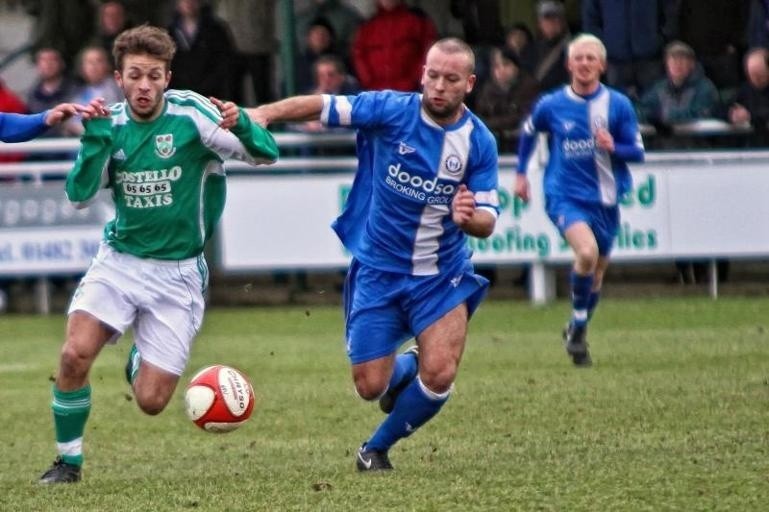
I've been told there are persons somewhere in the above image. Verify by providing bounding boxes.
[33,24,279,485]
[238,36,501,474]
[515,32,645,367]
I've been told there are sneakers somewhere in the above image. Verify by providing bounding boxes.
[565,327,587,358]
[379,346,419,413]
[355,441,394,472]
[126,343,139,383]
[563,322,593,366]
[39,456,82,485]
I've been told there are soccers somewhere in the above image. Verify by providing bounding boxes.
[186,366,254,432]
